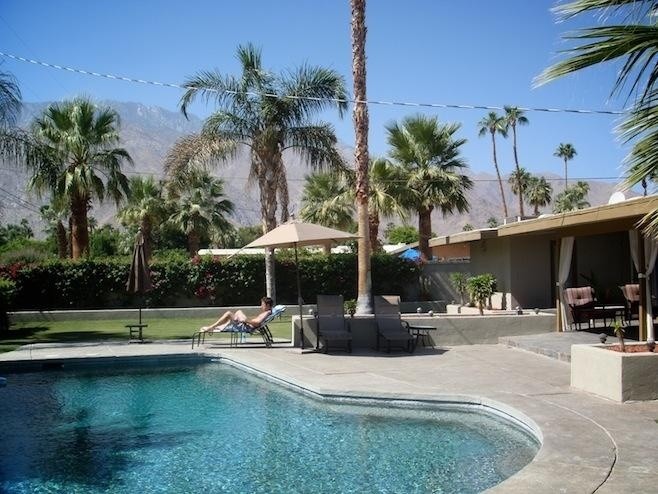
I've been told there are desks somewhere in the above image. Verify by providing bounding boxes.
[406,326,437,351]
[595,306,629,327]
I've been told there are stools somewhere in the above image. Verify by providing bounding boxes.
[125,324,148,344]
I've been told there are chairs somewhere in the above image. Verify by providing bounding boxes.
[565,286,616,331]
[373,295,414,356]
[312,295,352,354]
[192,305,286,349]
[619,284,656,320]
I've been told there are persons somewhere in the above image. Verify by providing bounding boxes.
[199,295,273,333]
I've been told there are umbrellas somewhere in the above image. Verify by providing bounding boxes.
[236,217,365,344]
[125,225,155,323]
